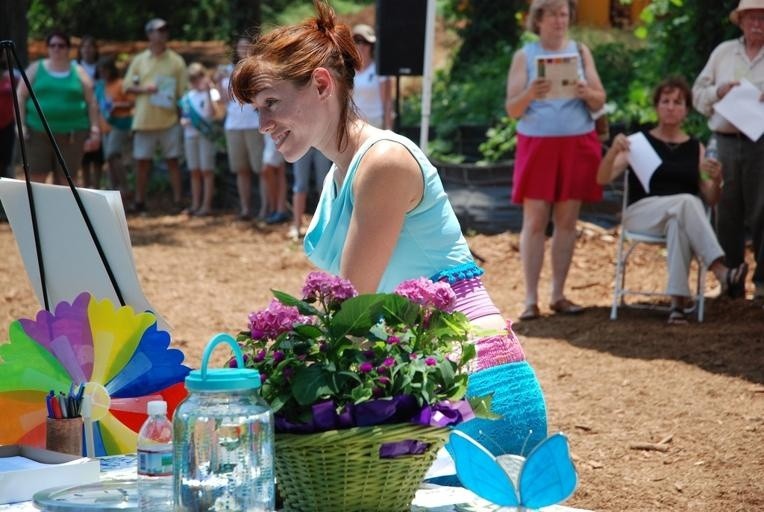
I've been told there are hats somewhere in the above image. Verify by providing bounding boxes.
[189,62,208,77]
[728,0,764,25]
[146,18,167,32]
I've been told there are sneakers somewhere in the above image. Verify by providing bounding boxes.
[128,201,146,215]
[239,209,290,224]
[287,225,302,238]
[168,203,183,217]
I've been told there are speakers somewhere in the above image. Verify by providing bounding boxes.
[375,0,428,76]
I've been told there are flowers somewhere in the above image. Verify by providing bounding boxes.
[222,270,502,457]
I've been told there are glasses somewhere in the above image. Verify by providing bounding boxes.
[48,43,67,49]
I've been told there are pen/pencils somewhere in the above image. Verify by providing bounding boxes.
[47,383,85,418]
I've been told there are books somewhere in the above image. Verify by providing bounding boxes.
[535,53,582,100]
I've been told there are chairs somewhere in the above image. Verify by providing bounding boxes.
[609,168,713,324]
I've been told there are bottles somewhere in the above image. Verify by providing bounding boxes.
[704,137,721,172]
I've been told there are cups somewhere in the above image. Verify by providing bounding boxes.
[136,402,174,511]
[45,415,84,458]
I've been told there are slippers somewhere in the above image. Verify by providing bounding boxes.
[668,307,689,328]
[519,304,543,321]
[549,298,584,314]
[720,262,747,297]
[182,206,212,217]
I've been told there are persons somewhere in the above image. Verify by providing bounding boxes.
[597,78,749,327]
[591,104,611,144]
[507,1,603,321]
[2,13,389,244]
[233,2,547,483]
[690,1,763,299]
